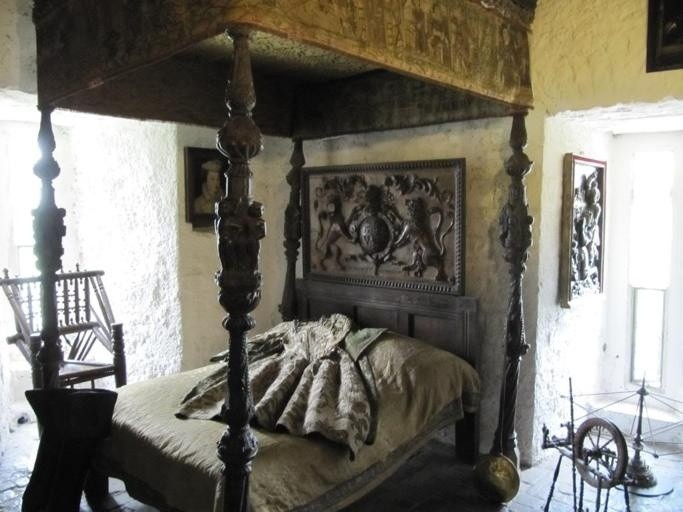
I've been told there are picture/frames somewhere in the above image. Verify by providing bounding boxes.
[183,146,229,222]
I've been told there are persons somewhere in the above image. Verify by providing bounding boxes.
[193,160,225,216]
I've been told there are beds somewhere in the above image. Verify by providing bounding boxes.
[107,278,479,512]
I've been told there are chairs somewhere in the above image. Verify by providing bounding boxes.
[0,263,126,390]
